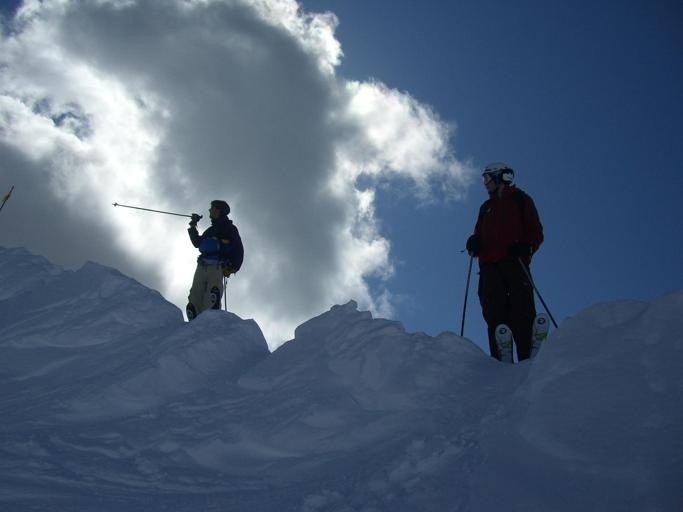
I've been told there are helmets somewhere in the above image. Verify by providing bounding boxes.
[212,200,230,215]
[482,162,514,184]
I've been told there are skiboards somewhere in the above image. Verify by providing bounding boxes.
[495,314,550,363]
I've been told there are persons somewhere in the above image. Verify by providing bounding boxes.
[186,200,244,322]
[466,162,545,361]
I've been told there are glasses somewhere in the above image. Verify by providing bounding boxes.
[484,167,507,184]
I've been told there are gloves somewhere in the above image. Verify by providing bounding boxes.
[517,241,532,258]
[467,236,482,254]
[224,269,232,276]
[189,214,200,226]
